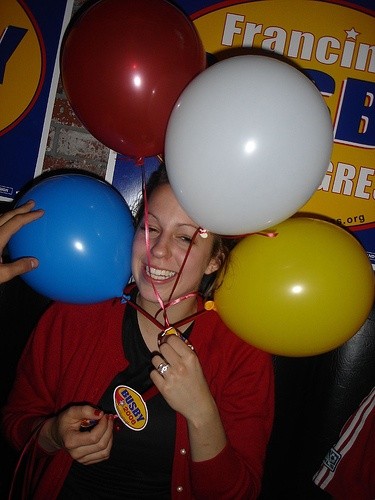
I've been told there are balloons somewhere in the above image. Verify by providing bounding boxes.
[60,0,206,165]
[164,54,333,236]
[8,173,136,304]
[203,217,375,357]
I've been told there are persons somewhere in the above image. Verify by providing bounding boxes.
[0,165,375,500]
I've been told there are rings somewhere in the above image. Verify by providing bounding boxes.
[158,362,170,374]
